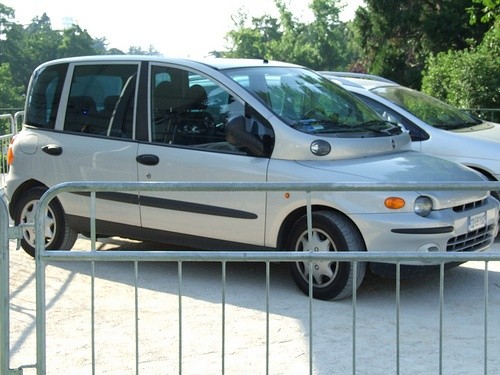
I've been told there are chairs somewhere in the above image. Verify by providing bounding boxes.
[183,84,213,145]
[68,96,101,134]
[154,81,183,145]
[99,95,119,125]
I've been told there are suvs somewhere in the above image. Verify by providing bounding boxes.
[4,54,500,301]
[173,69,500,204]
